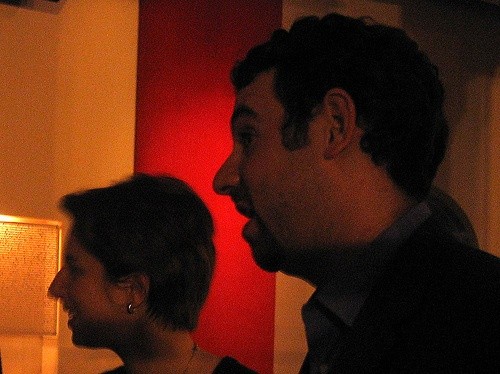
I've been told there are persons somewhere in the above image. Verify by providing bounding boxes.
[212,12,500,373]
[46,168,258,374]
